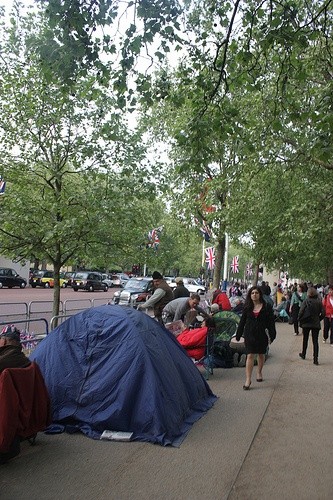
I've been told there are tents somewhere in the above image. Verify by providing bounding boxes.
[202,311,269,369]
[28,304,221,449]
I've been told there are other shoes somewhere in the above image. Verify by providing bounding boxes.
[323,338,326,343]
[294,332,298,336]
[314,360,318,365]
[257,379,262,382]
[299,352,305,359]
[243,380,251,390]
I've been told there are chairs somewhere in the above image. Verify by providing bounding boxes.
[274,301,287,323]
[186,326,217,381]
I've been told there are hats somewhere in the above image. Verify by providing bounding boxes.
[175,277,183,284]
[152,271,163,279]
[0,324,21,340]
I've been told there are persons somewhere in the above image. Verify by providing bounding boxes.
[233,299,243,312]
[212,289,232,311]
[297,288,326,365]
[236,286,276,389]
[322,285,333,345]
[262,280,331,336]
[0,331,32,375]
[136,271,216,359]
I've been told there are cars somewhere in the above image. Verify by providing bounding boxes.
[29,267,131,292]
[0,267,27,288]
[108,276,207,308]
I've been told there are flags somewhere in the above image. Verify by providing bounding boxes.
[205,247,215,270]
[202,221,220,241]
[146,228,164,251]
[246,262,254,276]
[230,255,240,274]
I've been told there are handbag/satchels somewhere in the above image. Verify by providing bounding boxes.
[300,301,310,323]
[289,314,294,325]
